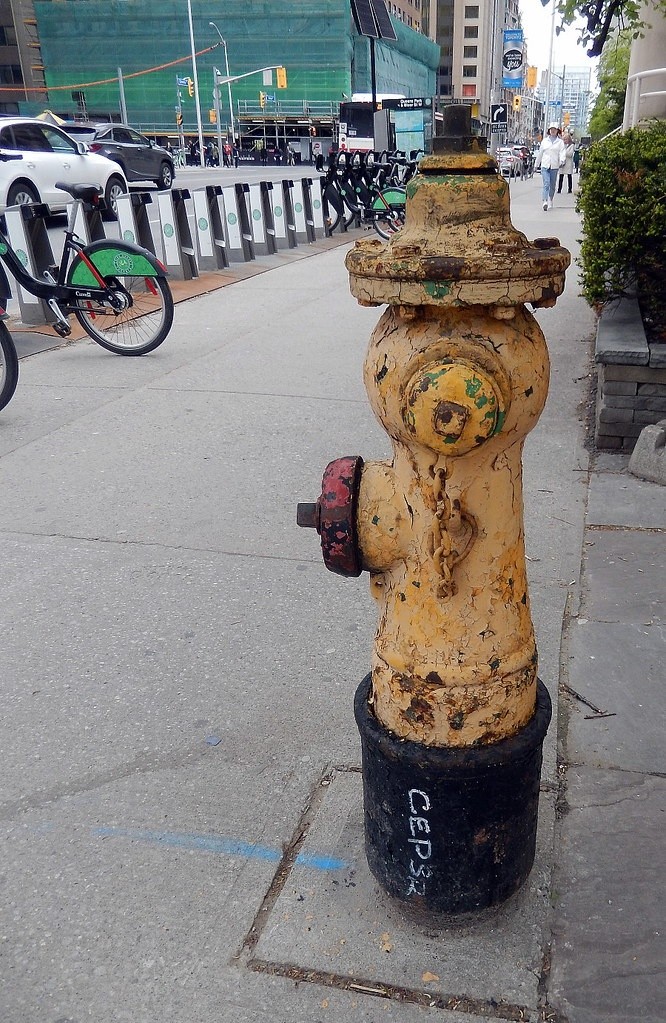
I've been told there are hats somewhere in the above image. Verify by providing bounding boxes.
[547,122,561,134]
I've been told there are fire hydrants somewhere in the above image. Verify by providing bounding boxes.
[293,101,568,915]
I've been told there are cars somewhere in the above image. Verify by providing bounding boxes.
[493,144,541,176]
[0,112,129,224]
[46,123,177,190]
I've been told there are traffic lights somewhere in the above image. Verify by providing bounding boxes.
[513,96,523,111]
[175,114,183,126]
[208,109,219,122]
[187,78,195,97]
[376,102,383,111]
[259,91,266,108]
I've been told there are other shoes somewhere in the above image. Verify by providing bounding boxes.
[548,202,553,208]
[542,202,548,212]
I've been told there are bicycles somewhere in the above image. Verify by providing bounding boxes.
[0,154,175,356]
[321,156,419,242]
[0,306,20,411]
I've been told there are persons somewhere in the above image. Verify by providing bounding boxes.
[313,146,319,164]
[521,147,529,180]
[557,133,586,194]
[203,141,240,168]
[188,139,198,166]
[261,144,297,166]
[534,124,566,211]
[165,141,173,154]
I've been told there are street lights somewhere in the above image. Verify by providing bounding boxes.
[209,21,237,144]
